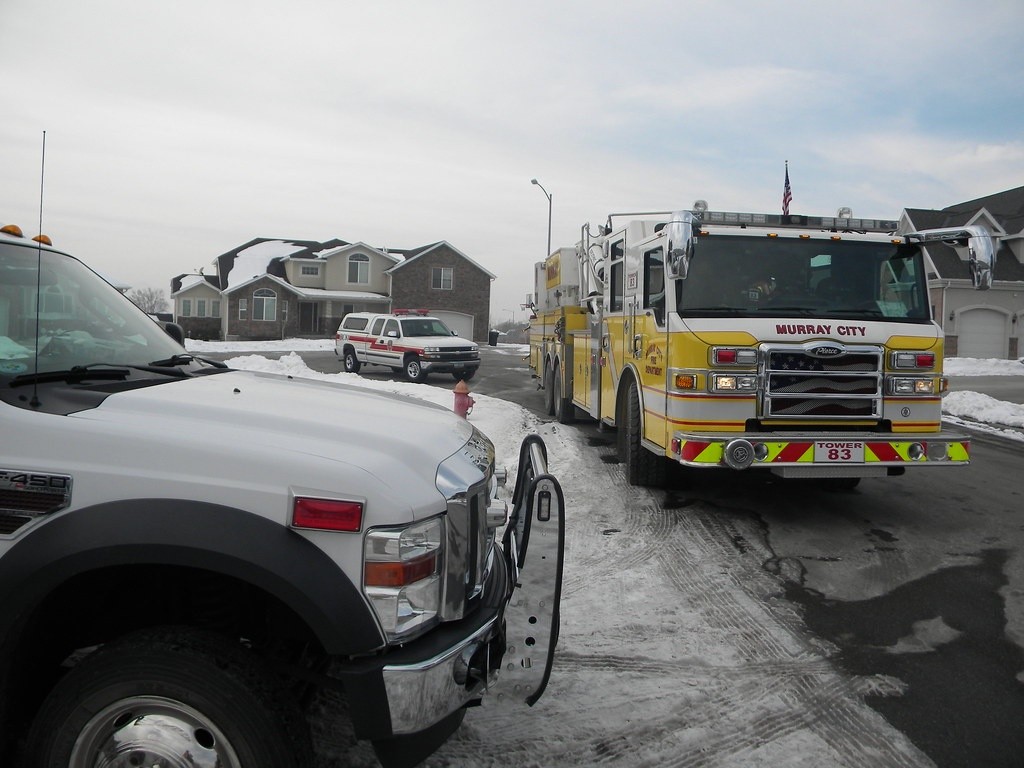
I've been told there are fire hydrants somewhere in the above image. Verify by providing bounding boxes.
[451,379,475,421]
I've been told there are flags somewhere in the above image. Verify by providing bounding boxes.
[783,164,793,216]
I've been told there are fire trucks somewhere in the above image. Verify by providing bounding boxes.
[529,201,970,488]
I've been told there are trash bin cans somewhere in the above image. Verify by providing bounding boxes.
[489,331,499,346]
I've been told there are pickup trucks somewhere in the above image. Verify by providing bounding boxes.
[335,306,480,383]
[0,224,564,768]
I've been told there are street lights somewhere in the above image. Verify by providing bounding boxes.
[531,178,553,257]
